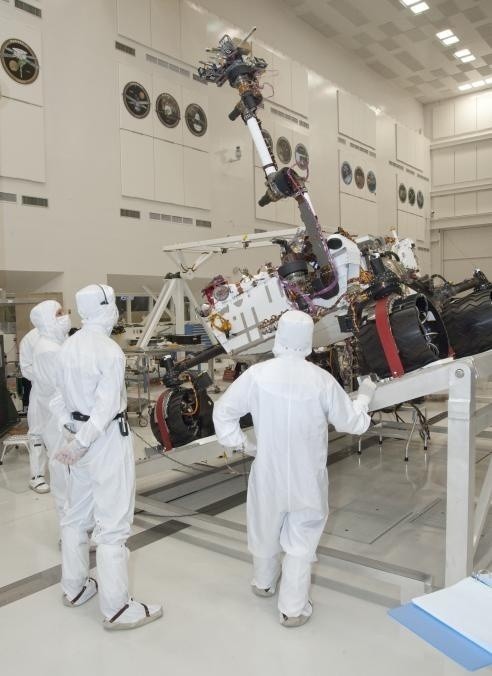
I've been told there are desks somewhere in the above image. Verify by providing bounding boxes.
[122,344,217,394]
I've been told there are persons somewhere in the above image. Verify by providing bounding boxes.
[212,308,377,627]
[56,283,164,630]
[29,300,99,553]
[18,327,50,494]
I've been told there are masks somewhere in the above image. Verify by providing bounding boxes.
[56,316,71,334]
